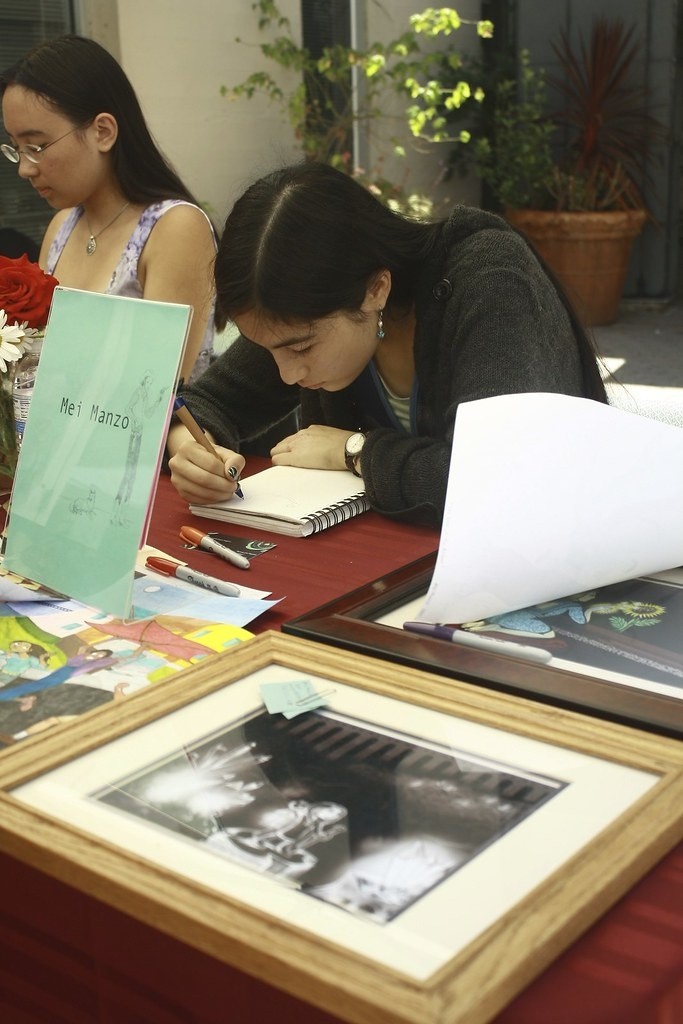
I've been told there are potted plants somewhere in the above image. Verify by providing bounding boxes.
[427,7,673,329]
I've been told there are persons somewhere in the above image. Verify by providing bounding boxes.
[0,36,219,385]
[159,162,610,532]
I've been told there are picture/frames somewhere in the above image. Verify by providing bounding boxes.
[280,548,683,742]
[1,629,683,1024]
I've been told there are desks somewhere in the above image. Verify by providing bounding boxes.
[0,453,683,1024]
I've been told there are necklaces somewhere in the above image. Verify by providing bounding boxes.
[86,201,132,256]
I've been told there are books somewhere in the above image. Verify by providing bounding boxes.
[188,465,373,538]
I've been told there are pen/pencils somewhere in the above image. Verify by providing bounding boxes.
[403,621,552,665]
[145,556,241,598]
[172,396,246,501]
[180,524,251,570]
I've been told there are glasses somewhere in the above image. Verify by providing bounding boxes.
[0,118,93,163]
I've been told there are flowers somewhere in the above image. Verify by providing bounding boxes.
[0,253,60,373]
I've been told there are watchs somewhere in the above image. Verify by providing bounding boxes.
[344,431,370,478]
